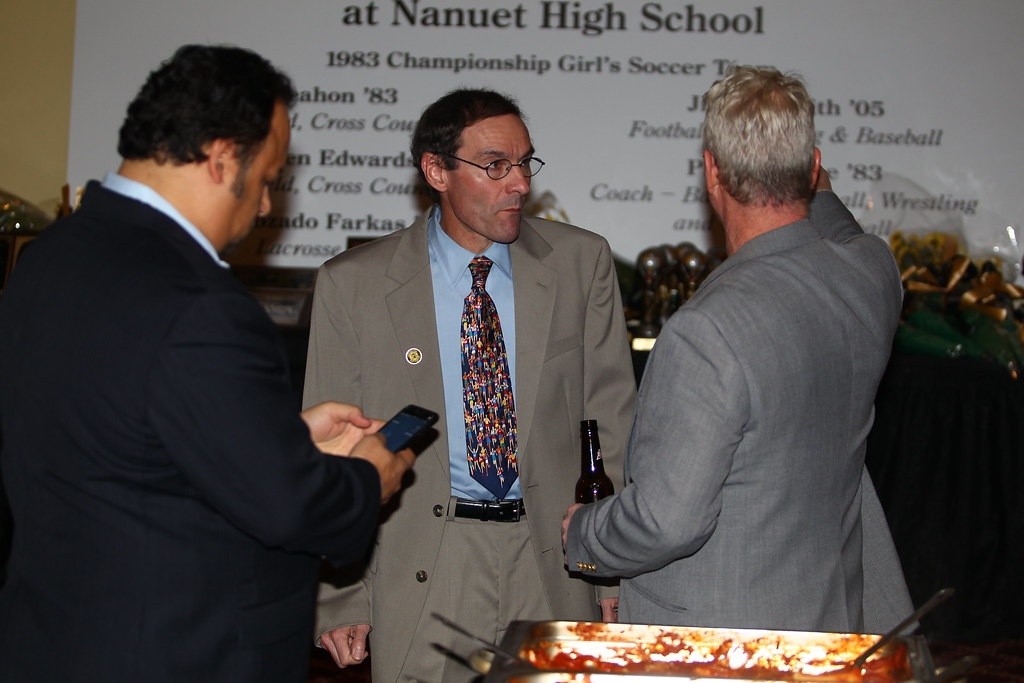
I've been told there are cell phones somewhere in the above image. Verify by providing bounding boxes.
[374,404,438,454]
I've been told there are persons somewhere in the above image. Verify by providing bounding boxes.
[0,44,417,683]
[560,67,921,637]
[302,89,638,683]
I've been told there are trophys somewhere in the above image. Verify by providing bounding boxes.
[634,241,707,344]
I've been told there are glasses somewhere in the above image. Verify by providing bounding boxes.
[424,150,546,181]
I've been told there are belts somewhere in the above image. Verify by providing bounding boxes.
[456,499,526,522]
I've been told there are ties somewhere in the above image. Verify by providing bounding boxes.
[460,256,518,499]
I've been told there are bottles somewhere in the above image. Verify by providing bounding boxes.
[0,202,28,232]
[575,420,615,506]
